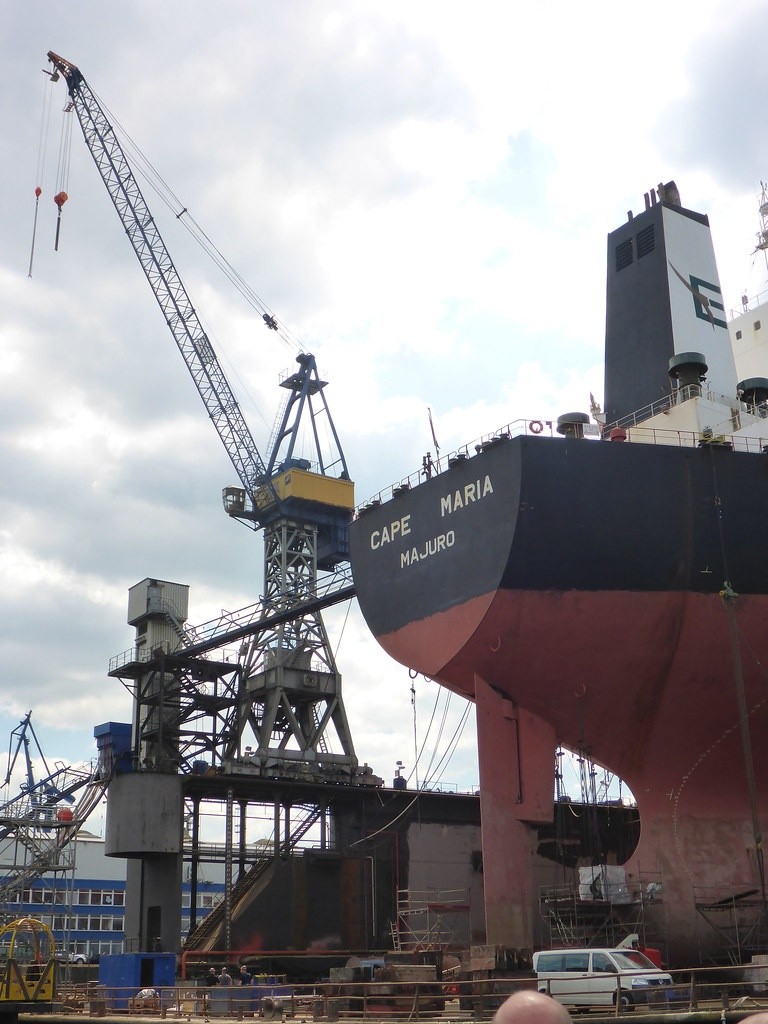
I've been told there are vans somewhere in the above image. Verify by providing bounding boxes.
[532,947,674,1013]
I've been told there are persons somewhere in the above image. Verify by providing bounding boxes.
[491,990,574,1024]
[737,1013,768,1024]
[238,965,253,986]
[205,968,221,987]
[217,967,233,987]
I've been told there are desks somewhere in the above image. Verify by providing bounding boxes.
[211,986,294,1013]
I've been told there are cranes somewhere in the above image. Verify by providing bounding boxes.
[27,50,383,973]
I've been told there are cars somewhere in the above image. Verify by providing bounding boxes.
[52,949,90,965]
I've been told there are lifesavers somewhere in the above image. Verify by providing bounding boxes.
[529,420,544,433]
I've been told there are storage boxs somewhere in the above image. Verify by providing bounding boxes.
[325,946,538,1018]
[196,1004,207,1013]
[252,973,287,986]
[183,1002,197,1014]
[648,987,698,1010]
[127,998,158,1008]
[193,760,208,774]
[90,1002,106,1017]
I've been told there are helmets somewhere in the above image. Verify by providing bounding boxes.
[210,968,216,972]
[221,967,227,971]
[240,965,247,970]
